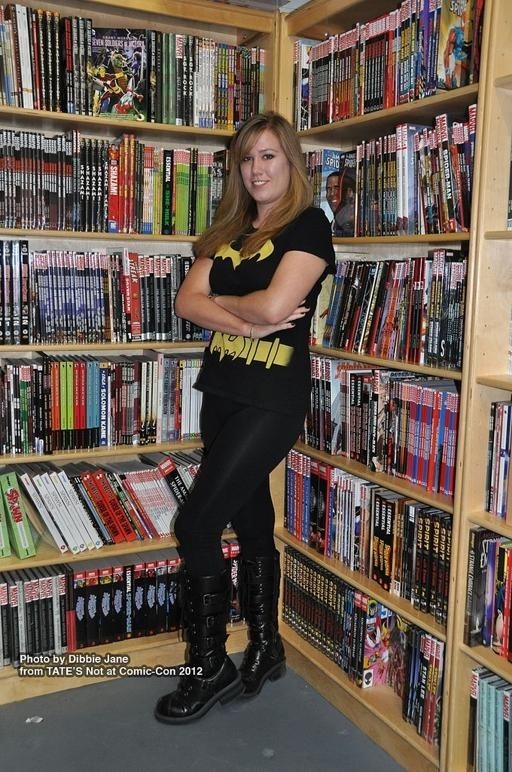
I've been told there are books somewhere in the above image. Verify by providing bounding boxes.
[0,2,510,770]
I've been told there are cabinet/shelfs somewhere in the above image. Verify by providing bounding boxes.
[279,0,491,772]
[444,0,512,771]
[0,0,278,708]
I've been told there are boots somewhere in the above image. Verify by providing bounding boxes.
[154,550,286,725]
